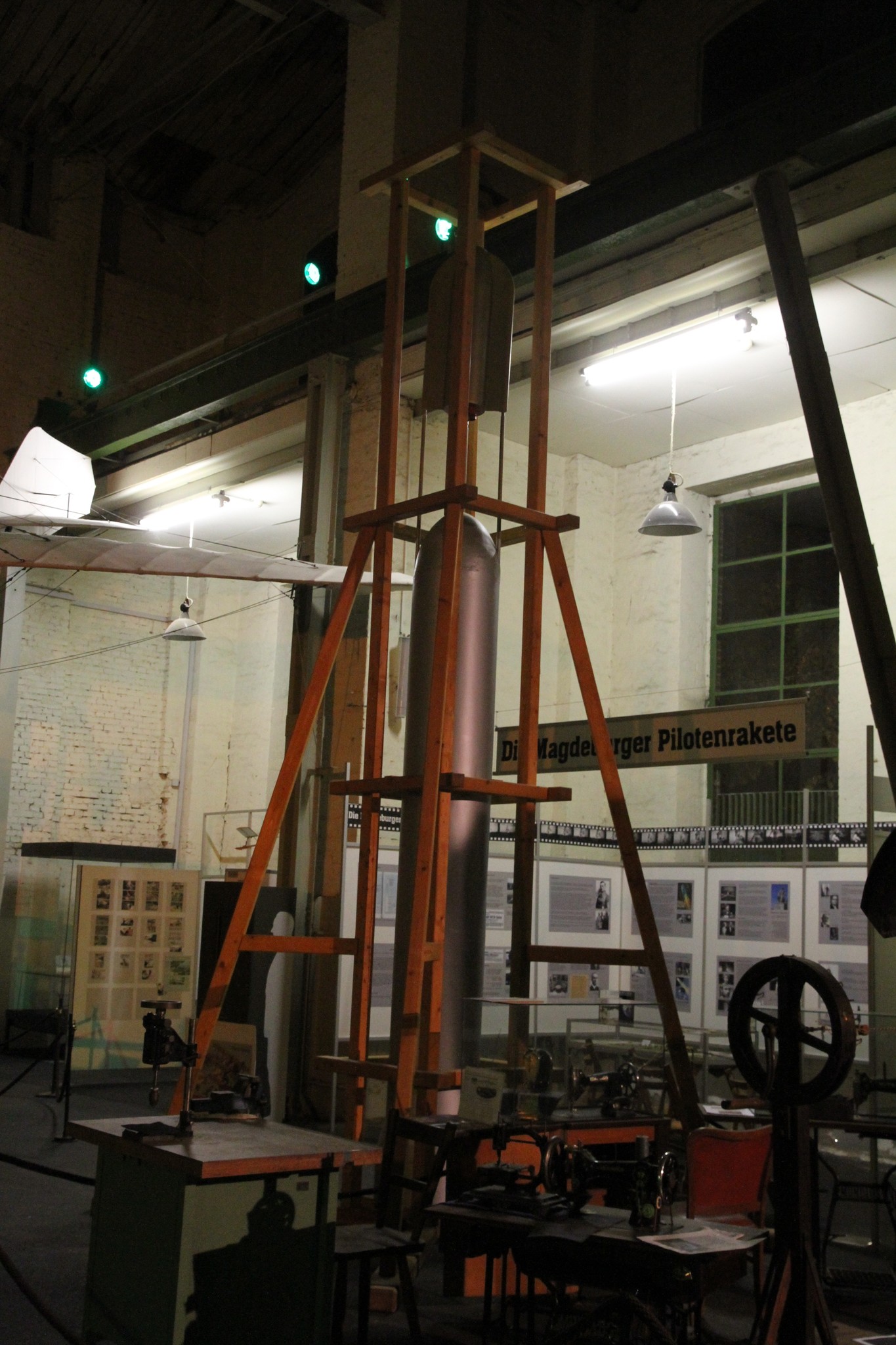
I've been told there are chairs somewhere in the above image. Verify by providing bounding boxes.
[333,1107,461,1345]
[658,1124,774,1345]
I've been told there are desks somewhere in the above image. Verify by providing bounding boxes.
[418,1112,671,1297]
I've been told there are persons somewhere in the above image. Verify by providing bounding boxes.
[264,911,294,1121]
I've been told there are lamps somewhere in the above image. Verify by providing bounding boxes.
[638,370,703,537]
[161,521,208,642]
